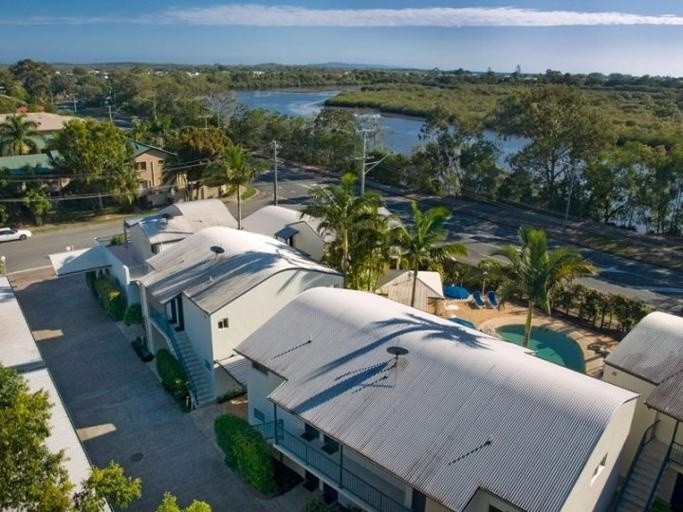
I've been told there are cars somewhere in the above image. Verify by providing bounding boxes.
[0,227,32,243]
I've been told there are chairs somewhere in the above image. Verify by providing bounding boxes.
[470,290,498,310]
